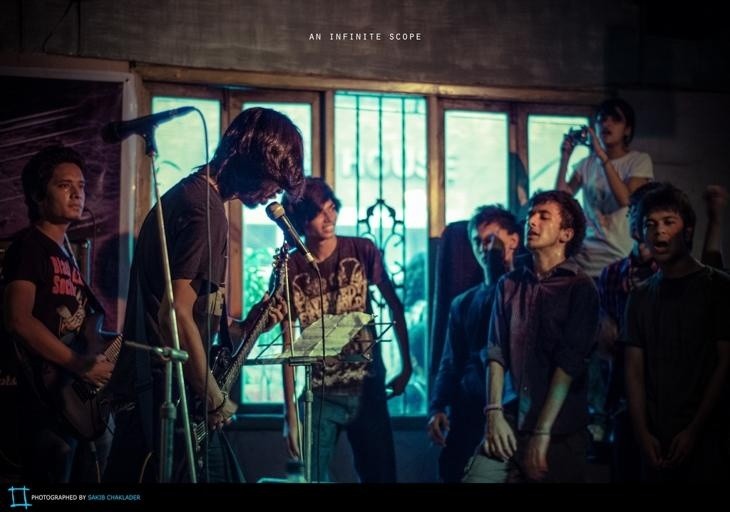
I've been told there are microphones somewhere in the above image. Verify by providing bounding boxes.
[265,201,321,272]
[100,105,193,145]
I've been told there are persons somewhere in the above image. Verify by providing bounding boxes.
[594,181,658,482]
[1,141,115,484]
[622,189,730,483]
[268,177,413,484]
[116,107,302,484]
[701,184,728,273]
[459,190,601,482]
[555,99,651,290]
[426,208,523,481]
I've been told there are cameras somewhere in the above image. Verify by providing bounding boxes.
[568,128,587,143]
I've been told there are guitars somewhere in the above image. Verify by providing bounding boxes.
[31,331,123,440]
[158,242,291,450]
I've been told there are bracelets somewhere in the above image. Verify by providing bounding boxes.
[483,403,504,414]
[533,429,552,438]
[206,390,229,413]
